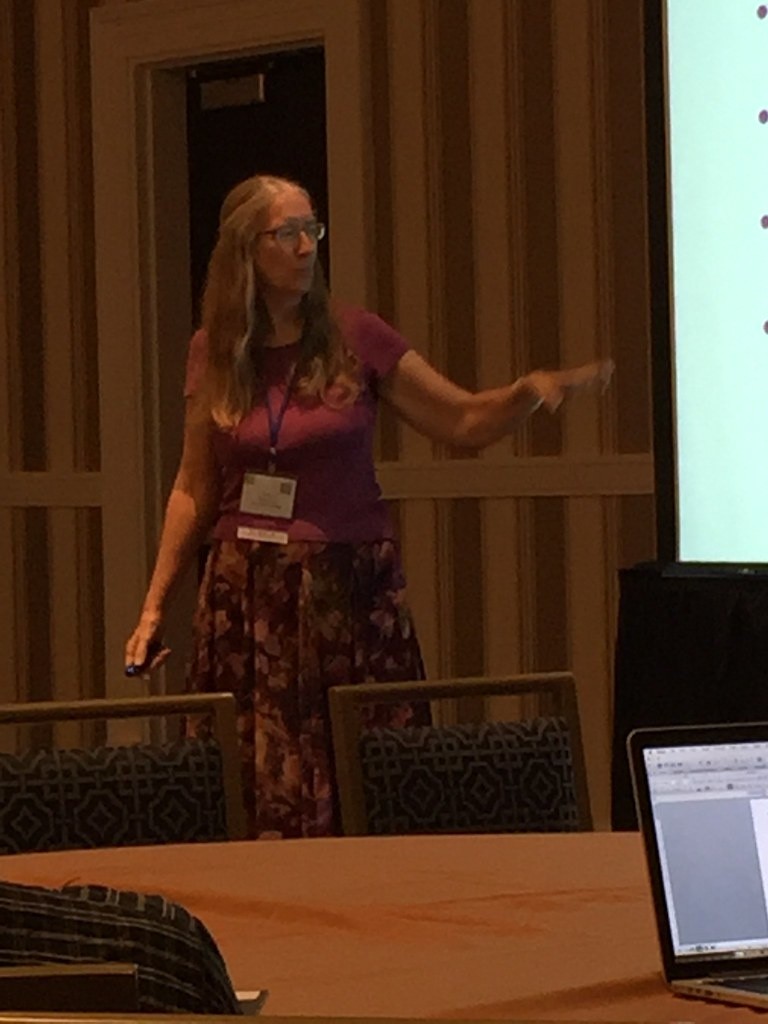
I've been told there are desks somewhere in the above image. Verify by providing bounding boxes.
[0,832,768,1024]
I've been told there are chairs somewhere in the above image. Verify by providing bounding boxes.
[328,673,594,838]
[0,694,243,856]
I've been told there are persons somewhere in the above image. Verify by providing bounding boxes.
[124,174,564,841]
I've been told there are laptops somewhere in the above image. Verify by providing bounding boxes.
[625,723,768,1013]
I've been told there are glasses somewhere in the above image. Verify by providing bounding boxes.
[259,221,326,247]
